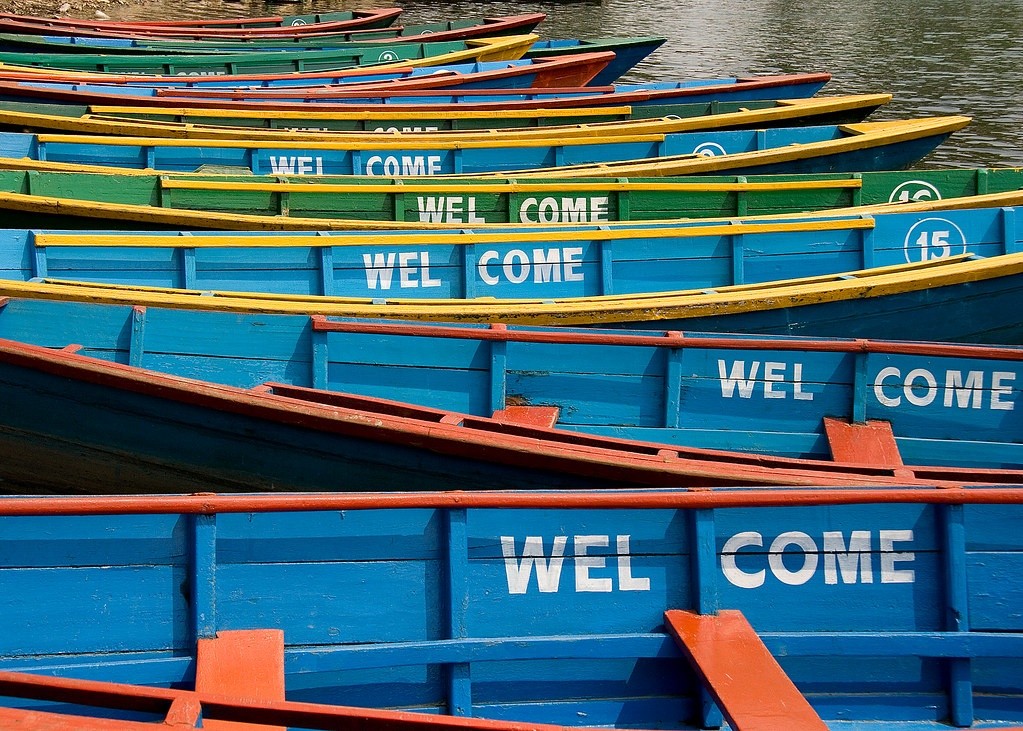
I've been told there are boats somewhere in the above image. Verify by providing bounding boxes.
[1,6,1023,731]
